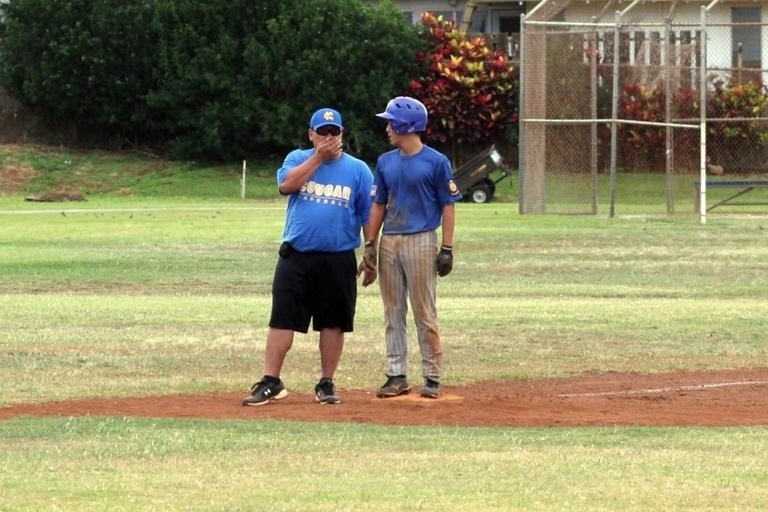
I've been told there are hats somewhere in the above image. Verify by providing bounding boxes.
[310,108,344,131]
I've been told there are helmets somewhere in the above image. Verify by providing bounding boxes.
[375,96,428,135]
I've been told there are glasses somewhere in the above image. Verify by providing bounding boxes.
[311,127,341,136]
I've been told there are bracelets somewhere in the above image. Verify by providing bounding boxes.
[364,240,375,248]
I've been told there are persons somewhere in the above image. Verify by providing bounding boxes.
[363,95,463,397]
[241,108,377,406]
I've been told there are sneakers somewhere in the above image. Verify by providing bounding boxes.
[420,379,439,397]
[315,383,340,405]
[243,380,289,406]
[376,375,411,396]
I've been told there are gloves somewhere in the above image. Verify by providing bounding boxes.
[435,245,453,277]
